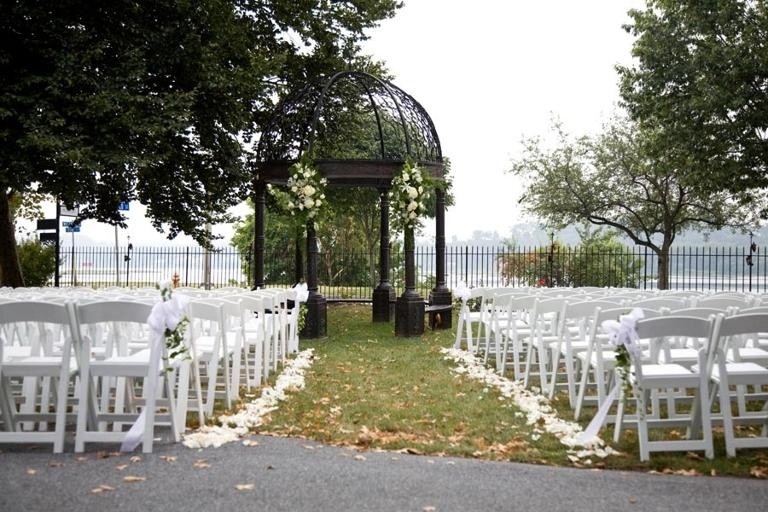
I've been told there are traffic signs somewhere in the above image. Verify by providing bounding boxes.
[119,202,129,211]
[66,227,79,232]
[62,222,82,227]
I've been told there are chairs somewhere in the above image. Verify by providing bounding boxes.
[454,286,768,461]
[0,284,300,454]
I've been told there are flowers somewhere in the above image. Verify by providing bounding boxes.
[385,159,431,234]
[267,158,329,237]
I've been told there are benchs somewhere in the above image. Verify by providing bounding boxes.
[327,297,451,313]
